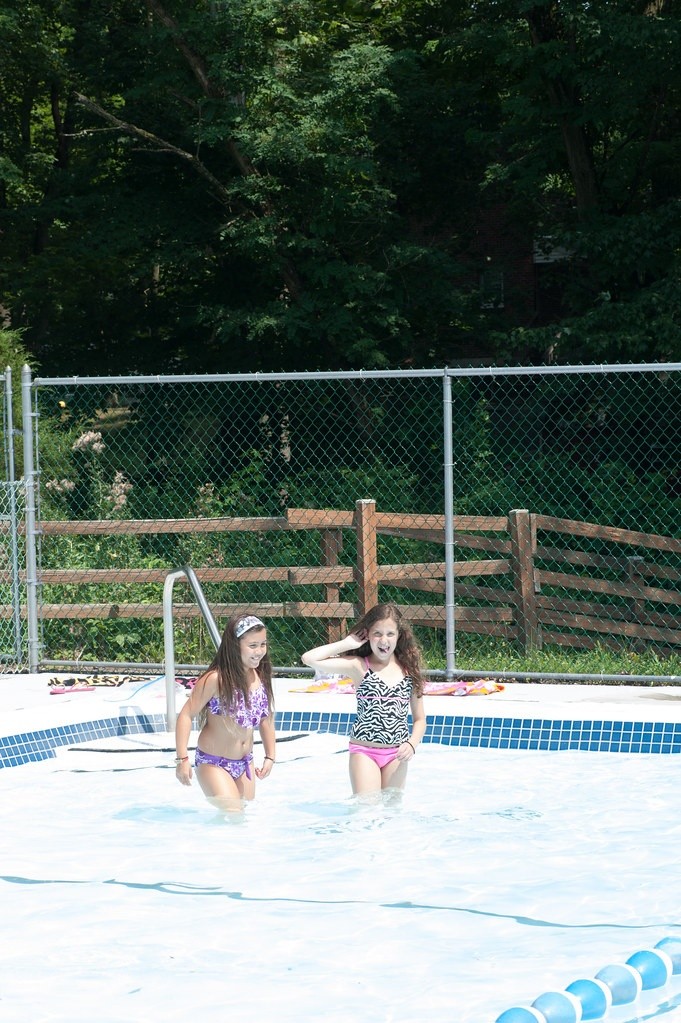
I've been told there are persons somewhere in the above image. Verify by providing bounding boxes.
[300,604,426,795]
[174,613,277,799]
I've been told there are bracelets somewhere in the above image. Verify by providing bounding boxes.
[175,756,189,764]
[264,756,275,763]
[404,741,416,754]
